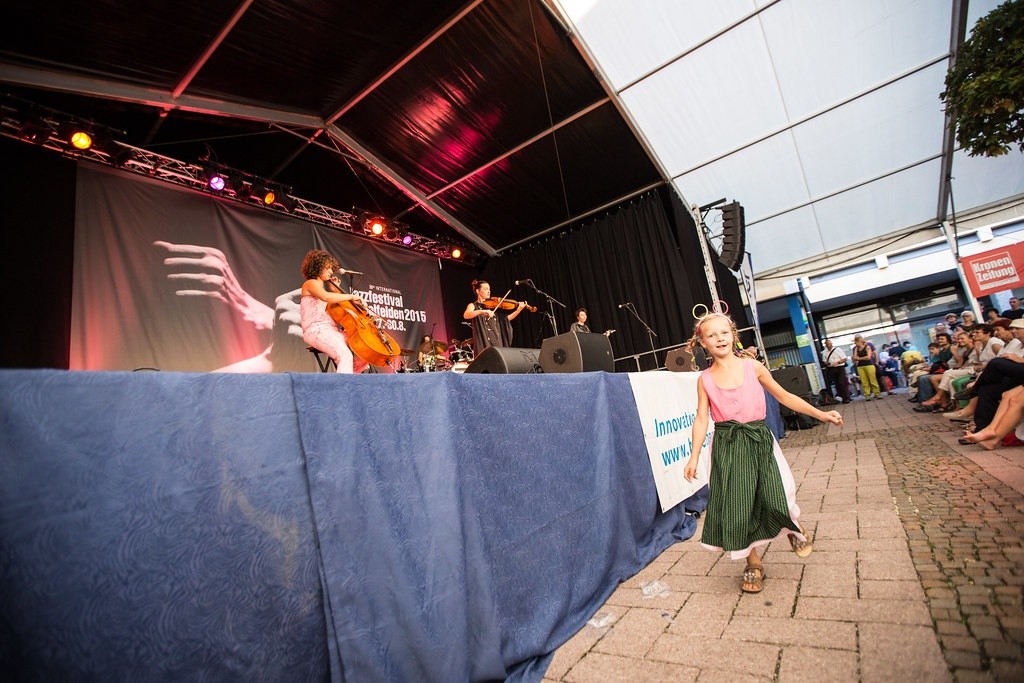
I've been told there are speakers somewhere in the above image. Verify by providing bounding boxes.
[771,366,809,396]
[464,346,543,374]
[719,201,745,272]
[664,346,708,372]
[538,331,615,374]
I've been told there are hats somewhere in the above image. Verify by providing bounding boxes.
[1009,319,1024,328]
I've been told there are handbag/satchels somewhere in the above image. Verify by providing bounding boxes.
[930,361,949,373]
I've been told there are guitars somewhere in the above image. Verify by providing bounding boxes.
[603,329,616,336]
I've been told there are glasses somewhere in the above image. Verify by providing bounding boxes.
[973,331,985,334]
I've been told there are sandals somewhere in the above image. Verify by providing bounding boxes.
[740,563,766,594]
[922,397,943,406]
[788,522,813,559]
[933,402,952,413]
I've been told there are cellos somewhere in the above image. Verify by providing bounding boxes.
[327,277,401,374]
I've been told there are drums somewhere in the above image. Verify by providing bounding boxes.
[448,350,474,363]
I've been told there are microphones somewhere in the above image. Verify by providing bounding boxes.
[462,322,471,325]
[338,268,363,275]
[619,303,630,308]
[515,279,530,285]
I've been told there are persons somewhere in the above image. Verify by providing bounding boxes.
[299,249,369,375]
[685,300,843,592]
[745,346,766,366]
[570,308,609,337]
[821,340,853,404]
[152,241,326,372]
[849,296,1024,451]
[417,336,443,373]
[463,279,527,358]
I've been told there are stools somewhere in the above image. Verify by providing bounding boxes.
[307,344,338,373]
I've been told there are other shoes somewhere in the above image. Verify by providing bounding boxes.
[913,405,934,413]
[877,396,883,399]
[865,397,871,401]
[888,392,897,395]
[841,399,853,404]
[908,397,919,403]
[942,411,1023,446]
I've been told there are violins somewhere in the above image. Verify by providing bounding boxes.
[483,296,538,313]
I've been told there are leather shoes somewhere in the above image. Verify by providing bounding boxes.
[954,388,976,400]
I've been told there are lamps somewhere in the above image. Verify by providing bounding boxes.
[450,243,462,258]
[399,229,411,245]
[349,211,385,236]
[13,104,138,167]
[228,170,300,214]
[203,164,227,191]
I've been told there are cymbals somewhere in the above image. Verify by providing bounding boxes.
[419,340,449,355]
[461,338,473,346]
[399,348,416,357]
[448,341,460,348]
